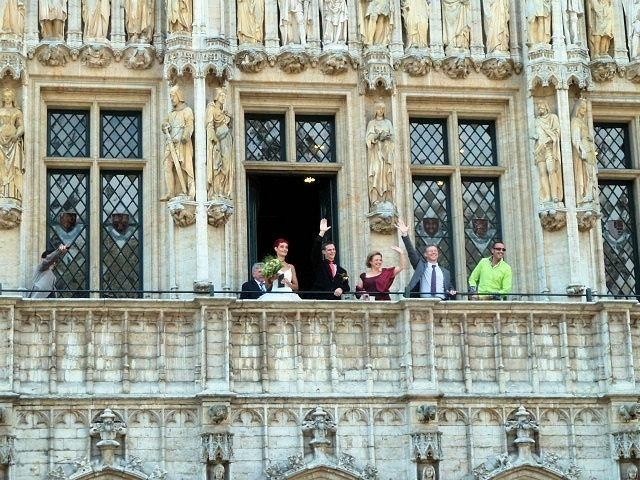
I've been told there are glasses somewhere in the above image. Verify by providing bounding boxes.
[494,248,506,252]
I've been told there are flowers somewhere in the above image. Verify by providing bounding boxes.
[259,254,286,281]
[341,273,349,282]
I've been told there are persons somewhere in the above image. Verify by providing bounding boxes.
[0,89,24,201]
[28,242,68,298]
[365,100,393,207]
[355,246,406,300]
[256,238,302,301]
[205,87,234,203]
[239,262,271,299]
[532,100,563,205]
[570,97,595,206]
[309,217,350,300]
[160,84,196,200]
[468,241,512,301]
[396,219,457,300]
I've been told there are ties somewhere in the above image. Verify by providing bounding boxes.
[330,261,336,277]
[431,265,436,296]
[259,282,266,292]
